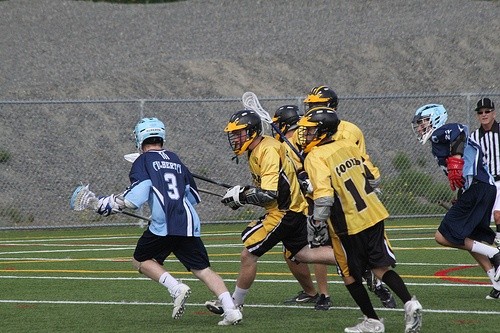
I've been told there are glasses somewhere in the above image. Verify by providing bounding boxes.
[477,110,493,114]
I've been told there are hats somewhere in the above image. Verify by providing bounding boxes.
[475,98,494,109]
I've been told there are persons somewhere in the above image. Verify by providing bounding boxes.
[296,108,423,333]
[96,118,243,325]
[411,104,500,300]
[205,86,372,333]
[457,98,500,251]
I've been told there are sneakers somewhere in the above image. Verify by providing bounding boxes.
[283,290,319,305]
[218,309,242,326]
[494,232,500,244]
[488,246,500,282]
[404,295,422,333]
[344,315,385,333]
[170,283,191,319]
[315,294,333,311]
[206,300,244,314]
[374,286,395,309]
[485,287,500,299]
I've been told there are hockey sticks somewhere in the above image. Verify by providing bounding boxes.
[438,201,500,246]
[124,154,233,188]
[241,92,303,164]
[195,185,224,198]
[71,186,149,221]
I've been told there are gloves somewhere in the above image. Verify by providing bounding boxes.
[307,215,329,249]
[96,194,117,217]
[446,157,466,191]
[221,185,245,210]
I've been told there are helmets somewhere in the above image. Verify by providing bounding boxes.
[132,117,165,152]
[224,110,262,155]
[410,104,448,145]
[296,107,341,153]
[303,86,339,113]
[272,105,301,133]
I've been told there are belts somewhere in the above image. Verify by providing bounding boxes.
[494,175,500,181]
